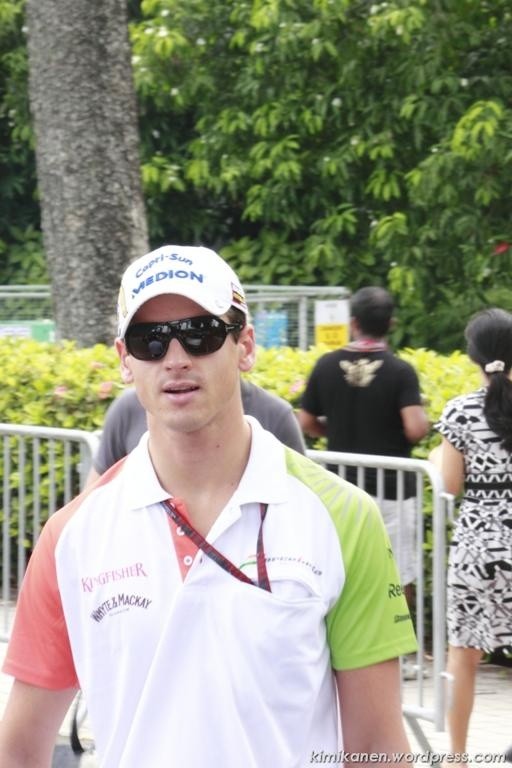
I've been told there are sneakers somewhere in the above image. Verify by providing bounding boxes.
[399,660,431,683]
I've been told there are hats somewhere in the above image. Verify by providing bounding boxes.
[114,245,248,341]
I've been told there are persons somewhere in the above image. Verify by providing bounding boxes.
[435,307,512,765]
[0,239,417,767]
[292,284,432,683]
[80,369,307,491]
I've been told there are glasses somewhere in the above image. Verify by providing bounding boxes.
[123,314,244,364]
